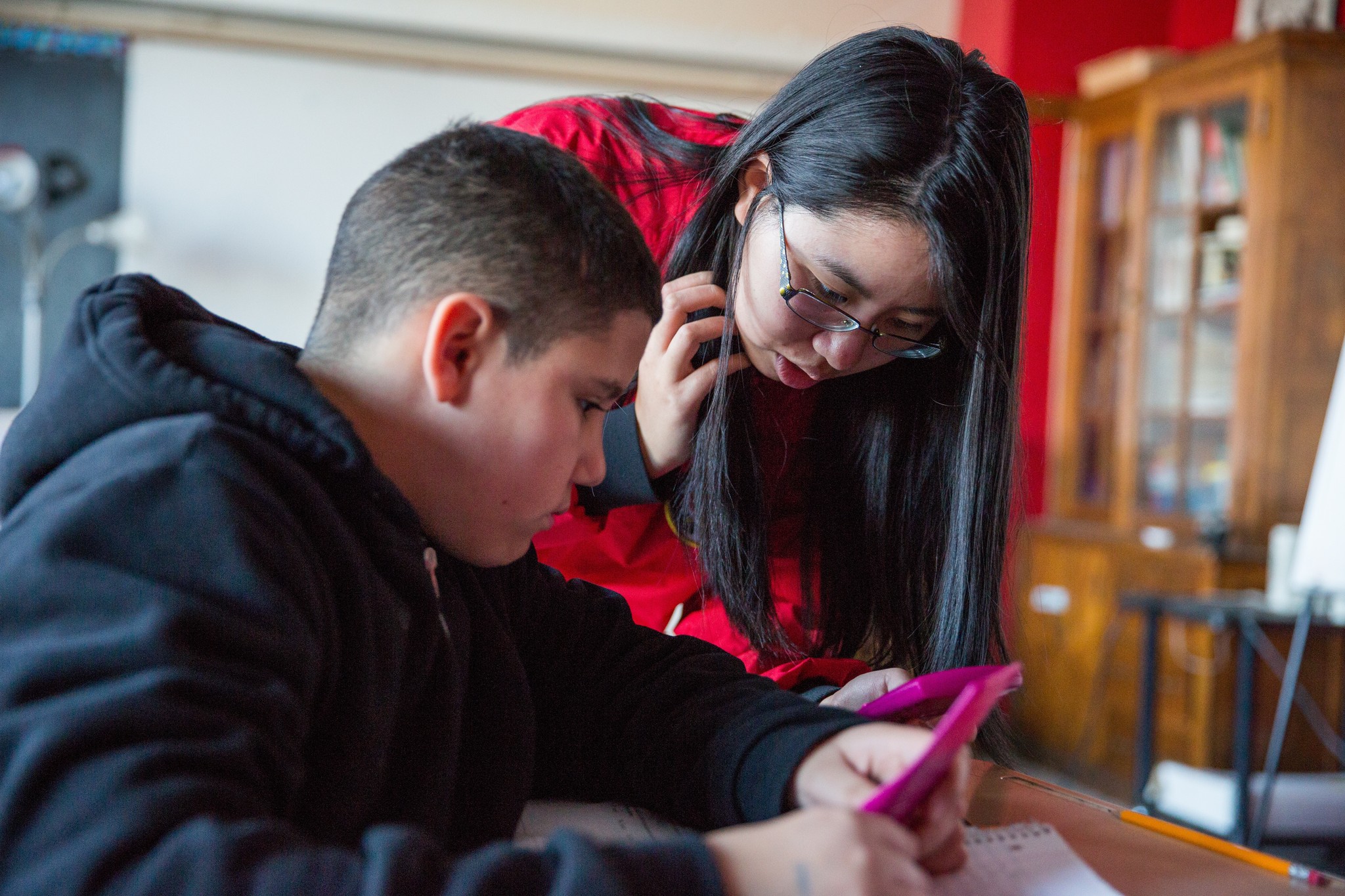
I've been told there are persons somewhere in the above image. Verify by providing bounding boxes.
[0,122,974,896]
[485,27,1034,767]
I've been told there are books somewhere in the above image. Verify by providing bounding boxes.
[934,820,1123,896]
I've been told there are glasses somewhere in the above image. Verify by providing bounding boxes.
[778,203,947,361]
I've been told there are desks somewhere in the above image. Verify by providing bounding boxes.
[1129,591,1345,846]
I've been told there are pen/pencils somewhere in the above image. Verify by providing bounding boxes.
[1108,809,1320,884]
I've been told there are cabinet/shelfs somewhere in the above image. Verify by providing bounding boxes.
[1008,25,1345,797]
